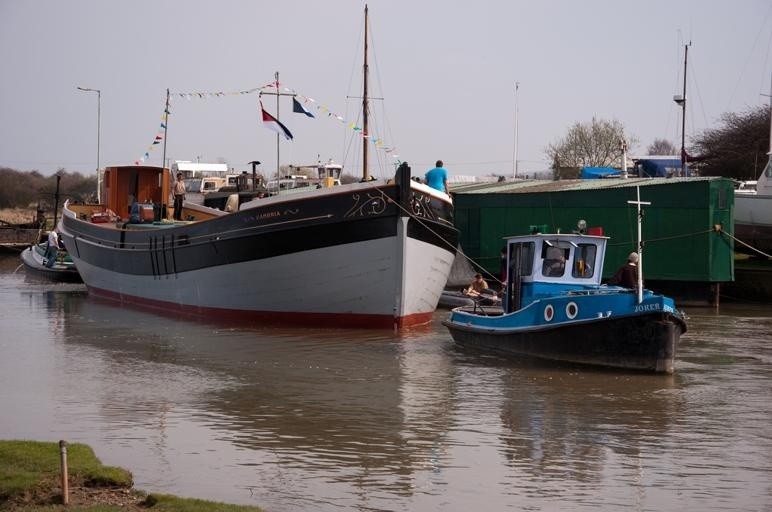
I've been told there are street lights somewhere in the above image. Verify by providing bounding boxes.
[78,86,99,202]
[513,83,519,178]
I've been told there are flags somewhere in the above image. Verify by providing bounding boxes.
[293,98,317,118]
[258,98,294,143]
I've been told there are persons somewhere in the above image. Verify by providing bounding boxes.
[423,159,454,218]
[170,173,186,221]
[500,248,513,315]
[252,192,264,200]
[465,273,488,295]
[604,251,645,289]
